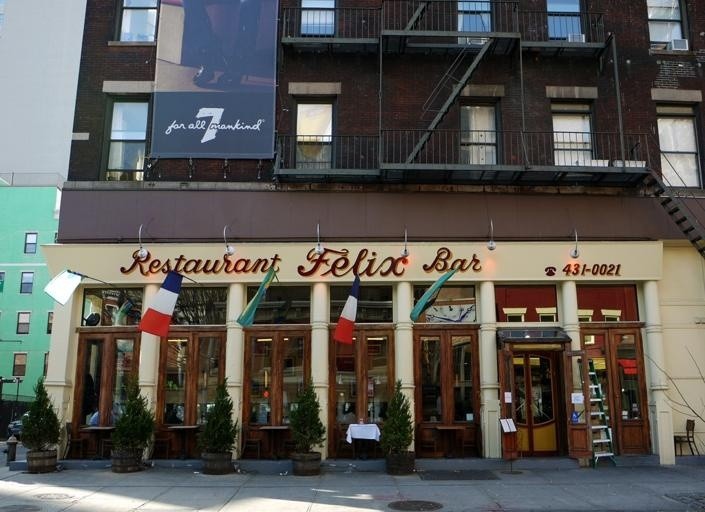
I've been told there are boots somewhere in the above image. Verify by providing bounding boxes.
[181,0,263,89]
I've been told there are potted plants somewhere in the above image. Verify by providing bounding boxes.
[195,376,239,473]
[19,376,63,473]
[286,374,326,475]
[107,370,155,472]
[380,380,416,474]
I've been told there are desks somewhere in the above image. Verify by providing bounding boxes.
[437,424,466,456]
[168,425,201,459]
[82,427,115,460]
[259,425,290,460]
[346,423,381,458]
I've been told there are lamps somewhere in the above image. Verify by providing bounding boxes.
[401,228,409,257]
[223,225,233,255]
[136,224,148,258]
[570,226,580,258]
[488,216,496,250]
[315,224,324,254]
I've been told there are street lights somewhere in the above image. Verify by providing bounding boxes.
[13,377,19,422]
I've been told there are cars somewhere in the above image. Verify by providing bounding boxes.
[7,420,25,440]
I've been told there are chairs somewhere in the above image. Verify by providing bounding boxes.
[241,421,261,459]
[154,426,172,459]
[99,437,112,457]
[674,419,700,457]
[333,421,350,460]
[62,423,89,459]
[284,439,297,452]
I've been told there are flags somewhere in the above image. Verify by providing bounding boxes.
[409,265,464,323]
[237,269,276,331]
[43,272,84,306]
[332,276,365,347]
[138,273,187,338]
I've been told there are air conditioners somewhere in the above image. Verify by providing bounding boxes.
[467,36,488,45]
[568,34,585,44]
[666,39,689,51]
